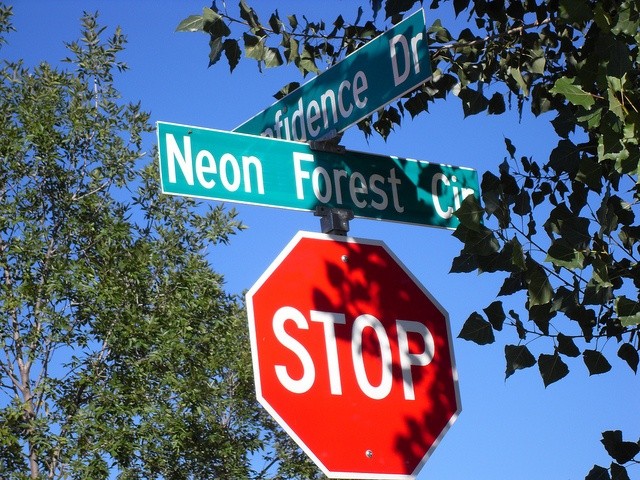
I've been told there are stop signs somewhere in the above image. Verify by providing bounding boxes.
[245,230,463,480]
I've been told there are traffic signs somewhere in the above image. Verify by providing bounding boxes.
[157,120,483,236]
[230,6,437,142]
